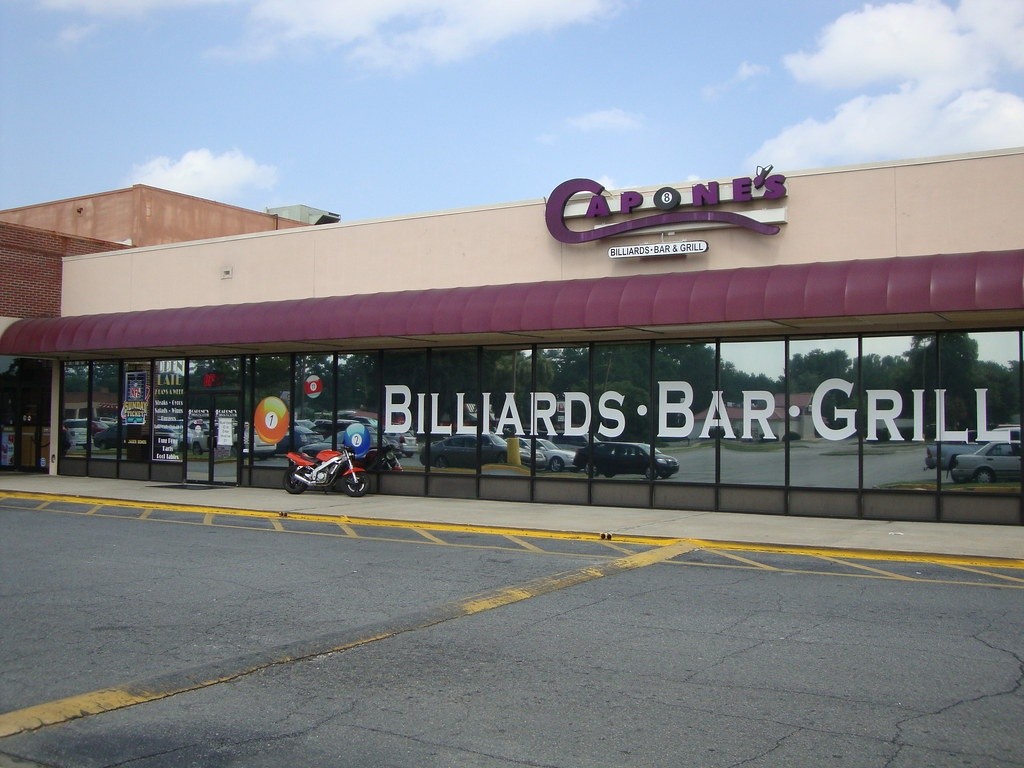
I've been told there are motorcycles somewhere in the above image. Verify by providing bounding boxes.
[282,438,371,498]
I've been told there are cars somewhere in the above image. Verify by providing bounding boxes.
[573,442,679,480]
[952,440,1023,484]
[502,437,547,467]
[155,416,418,471]
[62,416,148,451]
[525,438,578,474]
[925,424,1023,472]
[419,433,547,473]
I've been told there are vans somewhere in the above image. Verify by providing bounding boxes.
[545,433,604,454]
